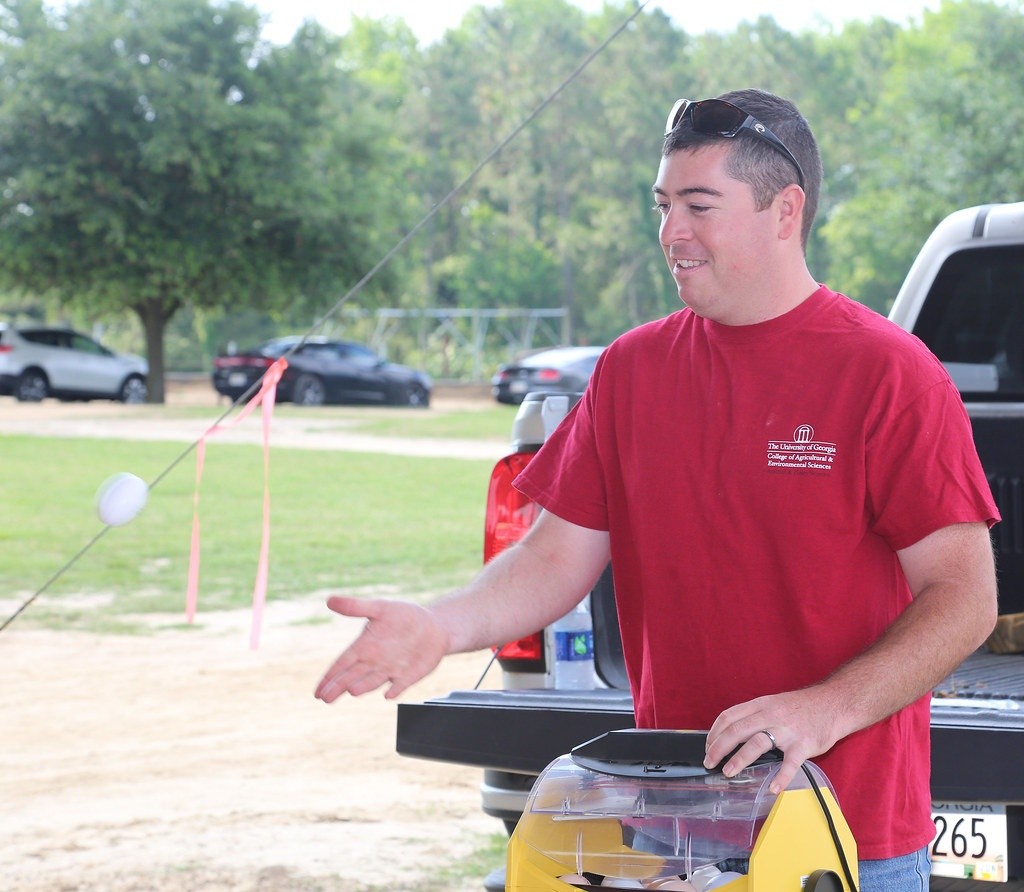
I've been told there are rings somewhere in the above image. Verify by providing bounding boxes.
[762,729,776,750]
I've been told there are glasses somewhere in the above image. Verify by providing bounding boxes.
[665,98,806,191]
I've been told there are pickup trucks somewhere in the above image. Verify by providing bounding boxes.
[386,203,1024,892]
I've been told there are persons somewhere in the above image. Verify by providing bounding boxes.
[315,89,1001,892]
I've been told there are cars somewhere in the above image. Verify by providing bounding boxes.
[0,324,150,406]
[213,333,436,409]
[494,345,604,406]
